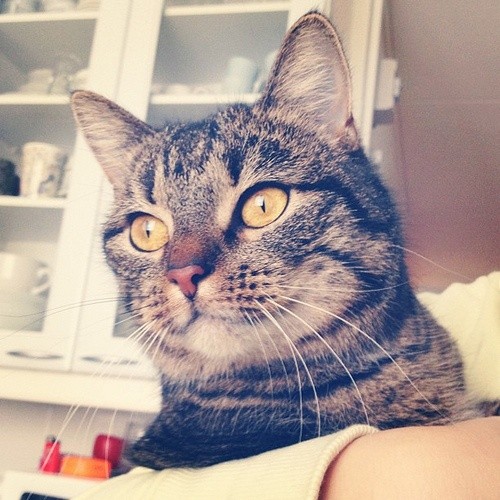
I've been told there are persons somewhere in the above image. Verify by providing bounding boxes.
[61,270,500,500]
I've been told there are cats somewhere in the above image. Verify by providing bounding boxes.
[28,5,499,500]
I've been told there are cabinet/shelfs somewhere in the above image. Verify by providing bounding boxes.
[0,0,383,413]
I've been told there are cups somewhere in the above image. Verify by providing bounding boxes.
[1,251,54,329]
[19,142,69,197]
[222,57,258,93]
